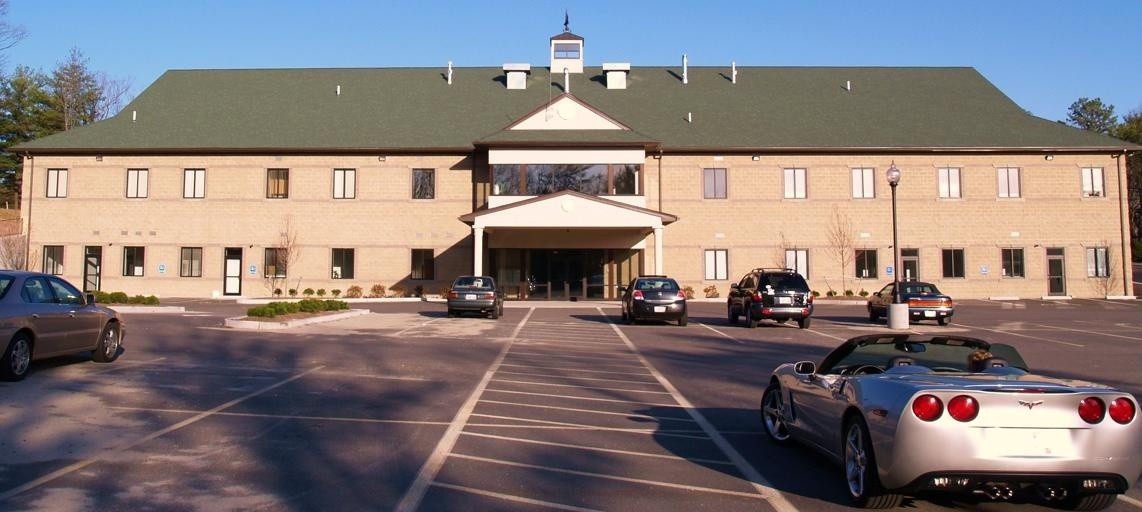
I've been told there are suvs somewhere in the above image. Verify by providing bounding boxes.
[724,268,816,330]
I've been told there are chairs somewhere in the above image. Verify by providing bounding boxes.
[885,356,1008,370]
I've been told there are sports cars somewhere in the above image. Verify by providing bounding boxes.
[760,326,1141,512]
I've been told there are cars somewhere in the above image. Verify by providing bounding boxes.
[446,275,503,321]
[1,267,126,381]
[866,279,955,327]
[615,273,689,328]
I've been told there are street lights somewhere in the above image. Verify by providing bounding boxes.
[886,160,907,305]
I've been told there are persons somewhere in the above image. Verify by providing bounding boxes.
[967,349,994,372]
